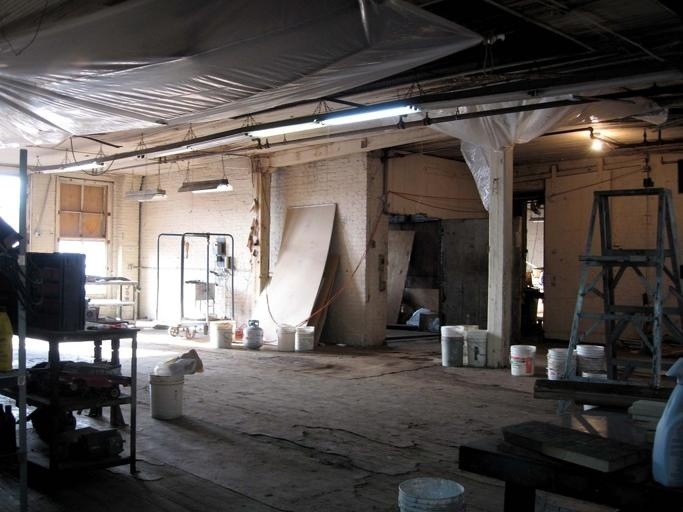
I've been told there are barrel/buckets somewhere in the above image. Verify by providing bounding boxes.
[294,326,314,351]
[467,329,487,367]
[441,326,464,366]
[577,344,608,379]
[149,374,184,419]
[464,324,478,364]
[214,323,232,347]
[397,477,466,511]
[511,345,537,377]
[277,325,295,352]
[546,347,577,380]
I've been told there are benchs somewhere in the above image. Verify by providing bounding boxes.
[459,434,681,511]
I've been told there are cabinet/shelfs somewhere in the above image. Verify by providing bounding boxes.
[86,280,138,326]
[0,322,141,496]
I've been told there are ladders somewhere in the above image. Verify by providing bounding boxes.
[562,188,683,389]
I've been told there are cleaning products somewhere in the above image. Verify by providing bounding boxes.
[0,312,12,372]
[653,357,682,486]
